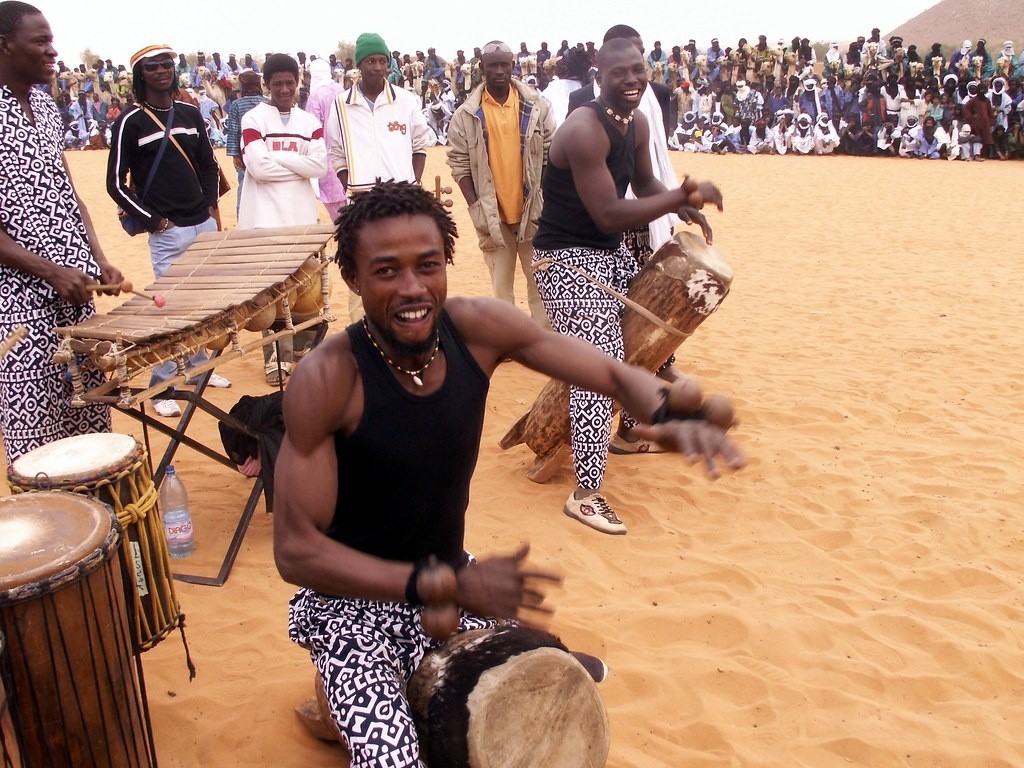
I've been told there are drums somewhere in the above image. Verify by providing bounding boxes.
[5,432,186,657]
[306,621,612,767]
[0,484,162,768]
[495,230,735,483]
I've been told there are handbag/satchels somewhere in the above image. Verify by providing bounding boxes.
[117,190,147,237]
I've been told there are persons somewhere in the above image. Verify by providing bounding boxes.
[0,0,126,468]
[226,24,723,534]
[273,178,746,768]
[37,40,600,152]
[646,28,1024,162]
[106,40,232,417]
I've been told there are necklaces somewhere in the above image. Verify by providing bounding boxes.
[604,105,634,125]
[362,317,440,387]
[145,100,174,111]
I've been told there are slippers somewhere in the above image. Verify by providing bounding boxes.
[267,369,286,386]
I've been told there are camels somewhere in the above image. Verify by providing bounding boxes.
[50,68,134,110]
[178,66,365,128]
[400,55,563,104]
[653,43,1011,80]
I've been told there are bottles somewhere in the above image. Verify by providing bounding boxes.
[160,465,194,559]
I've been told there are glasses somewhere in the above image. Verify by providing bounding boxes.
[479,43,512,58]
[142,60,174,72]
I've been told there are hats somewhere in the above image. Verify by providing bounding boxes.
[354,33,390,67]
[652,29,1019,137]
[130,41,177,69]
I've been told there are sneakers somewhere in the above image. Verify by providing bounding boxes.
[609,433,667,454]
[152,397,182,417]
[563,490,628,535]
[205,374,232,388]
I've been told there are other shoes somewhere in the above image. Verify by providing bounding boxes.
[569,651,608,684]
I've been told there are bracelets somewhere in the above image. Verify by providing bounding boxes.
[405,562,424,605]
[159,218,168,233]
